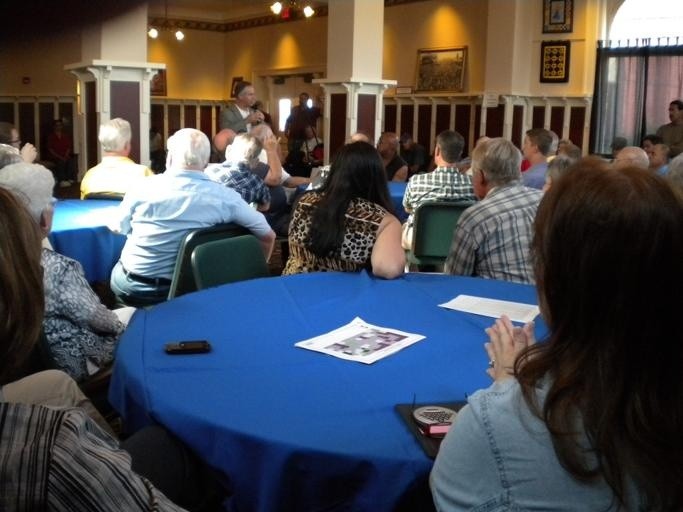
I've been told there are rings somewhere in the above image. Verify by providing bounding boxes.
[489,360,495,366]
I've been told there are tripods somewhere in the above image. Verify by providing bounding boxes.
[293,111,318,162]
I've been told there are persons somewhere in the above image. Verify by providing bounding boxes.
[1,125,126,382]
[111,128,276,309]
[429,160,683,511]
[81,82,682,286]
[0,187,183,511]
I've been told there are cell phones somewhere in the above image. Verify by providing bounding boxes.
[163,341,211,355]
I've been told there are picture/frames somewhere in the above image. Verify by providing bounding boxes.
[542,0,573,33]
[415,45,468,91]
[231,76,243,97]
[150,69,167,96]
[541,40,571,83]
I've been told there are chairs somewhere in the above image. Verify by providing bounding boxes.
[405,200,470,267]
[191,235,273,290]
[167,227,250,298]
[83,190,124,203]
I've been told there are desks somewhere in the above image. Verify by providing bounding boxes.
[49,198,126,284]
[297,181,409,225]
[109,270,551,511]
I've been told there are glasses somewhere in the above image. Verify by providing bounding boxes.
[411,392,468,438]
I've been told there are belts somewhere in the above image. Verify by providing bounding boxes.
[122,266,171,287]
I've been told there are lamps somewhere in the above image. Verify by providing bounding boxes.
[270,0,314,19]
[148,0,184,40]
